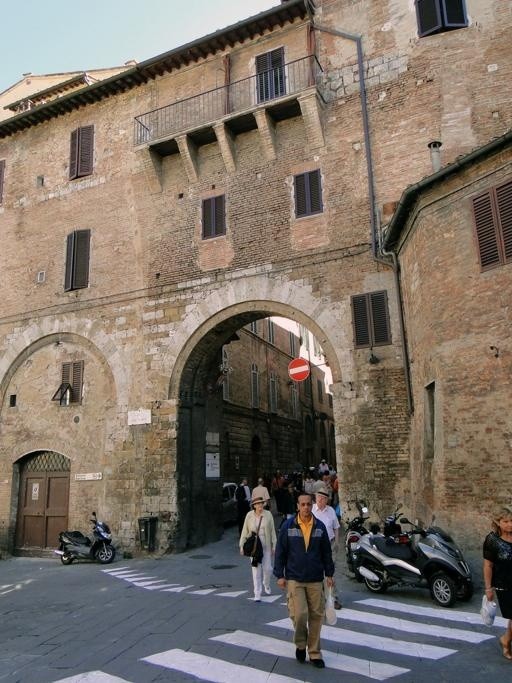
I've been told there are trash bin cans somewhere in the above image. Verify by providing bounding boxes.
[138,517,158,551]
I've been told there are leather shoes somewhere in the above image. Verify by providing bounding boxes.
[321,585,338,626]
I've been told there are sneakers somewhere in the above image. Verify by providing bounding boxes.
[295,644,307,665]
[310,652,326,674]
[335,600,342,610]
[254,593,261,602]
[263,582,272,595]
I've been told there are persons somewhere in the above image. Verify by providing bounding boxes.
[239,497,277,602]
[483,505,512,660]
[311,487,342,609]
[234,459,340,536]
[273,493,334,667]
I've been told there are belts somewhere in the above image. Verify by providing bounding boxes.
[330,536,335,542]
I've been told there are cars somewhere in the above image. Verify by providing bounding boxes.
[219,481,240,523]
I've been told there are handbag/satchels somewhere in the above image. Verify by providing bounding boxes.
[245,534,263,558]
[478,595,498,628]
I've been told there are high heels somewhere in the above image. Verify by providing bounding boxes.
[498,636,512,661]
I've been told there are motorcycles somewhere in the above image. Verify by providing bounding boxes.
[338,498,371,583]
[374,503,404,536]
[55,511,115,564]
[351,513,474,606]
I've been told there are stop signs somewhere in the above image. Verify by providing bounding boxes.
[287,358,310,382]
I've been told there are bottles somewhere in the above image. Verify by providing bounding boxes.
[485,603,497,627]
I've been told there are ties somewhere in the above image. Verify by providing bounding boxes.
[313,487,331,498]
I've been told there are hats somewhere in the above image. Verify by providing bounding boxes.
[249,498,268,506]
